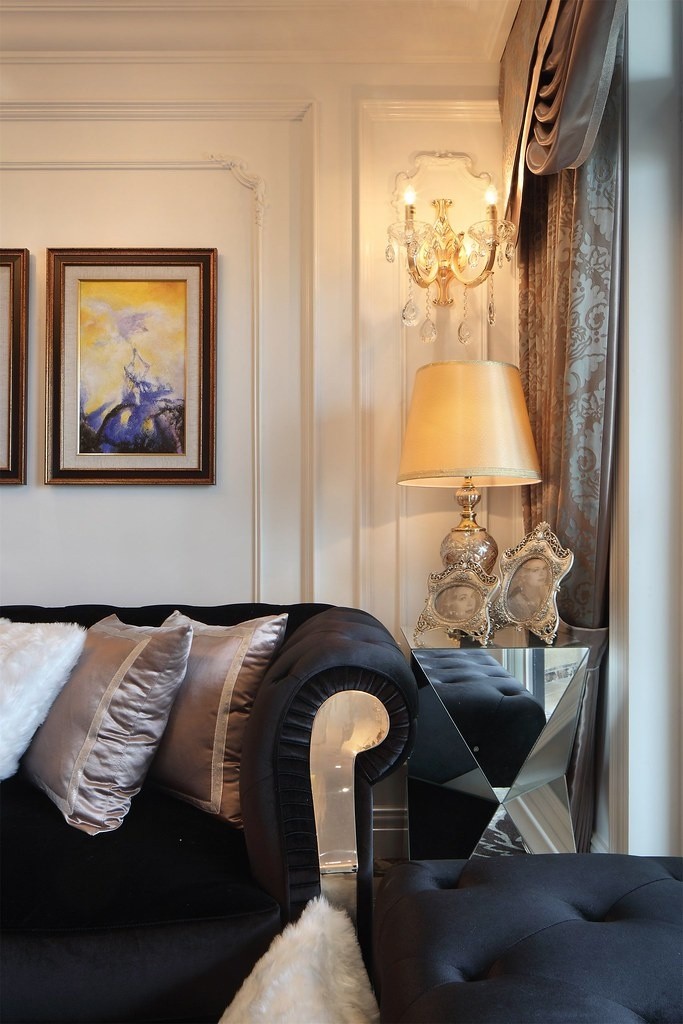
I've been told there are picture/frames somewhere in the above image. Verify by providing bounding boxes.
[0,247,29,487]
[43,247,216,488]
[486,519,575,647]
[413,552,501,647]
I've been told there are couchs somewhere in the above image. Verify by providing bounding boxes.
[2,595,499,1021]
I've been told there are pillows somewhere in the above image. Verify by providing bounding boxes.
[27,611,195,843]
[154,613,290,832]
[0,616,89,782]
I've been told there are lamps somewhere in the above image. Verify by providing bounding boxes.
[387,184,515,307]
[390,357,547,573]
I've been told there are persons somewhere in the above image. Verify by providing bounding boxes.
[507,559,550,621]
[435,585,481,621]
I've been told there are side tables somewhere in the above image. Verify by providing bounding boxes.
[394,622,591,861]
